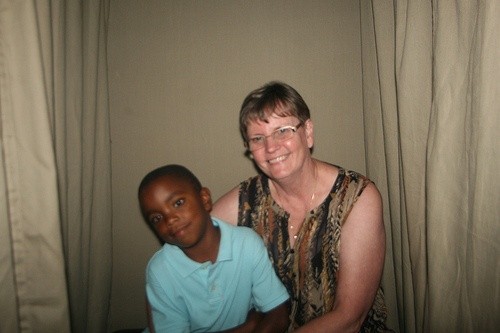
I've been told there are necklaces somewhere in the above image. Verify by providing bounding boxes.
[273,160,317,239]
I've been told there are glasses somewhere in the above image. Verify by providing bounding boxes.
[245,116,309,152]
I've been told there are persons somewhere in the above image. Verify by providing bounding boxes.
[138,164,292,333]
[144,80,394,333]
